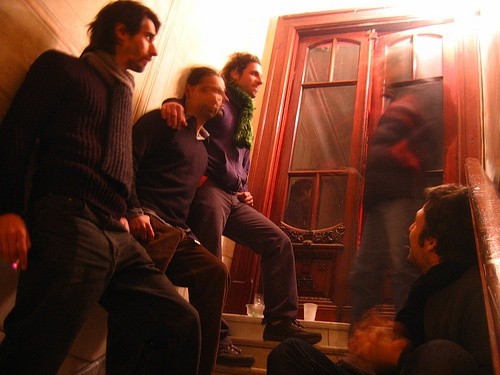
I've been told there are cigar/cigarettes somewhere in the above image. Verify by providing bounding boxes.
[11,258,20,271]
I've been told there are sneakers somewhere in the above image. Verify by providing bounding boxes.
[215,333,256,366]
[262,319,322,346]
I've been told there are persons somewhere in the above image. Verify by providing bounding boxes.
[160,50,323,366]
[349,181,495,375]
[125,65,229,375]
[284,177,313,226]
[265,337,482,375]
[351,48,434,320]
[0,0,202,375]
